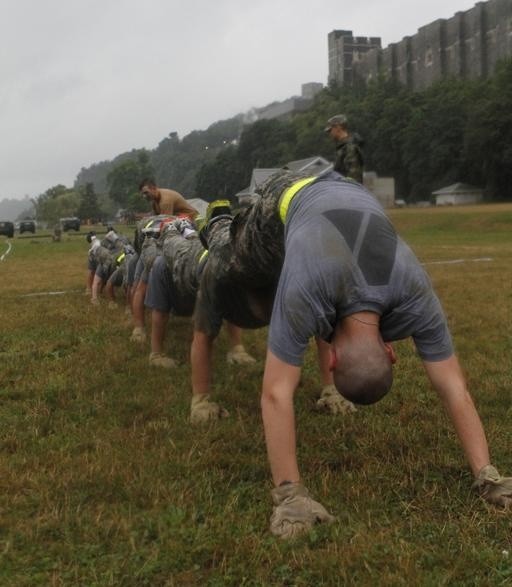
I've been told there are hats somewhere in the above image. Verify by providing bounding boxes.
[324,114,347,133]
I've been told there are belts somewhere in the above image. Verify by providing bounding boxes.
[148,351,181,370]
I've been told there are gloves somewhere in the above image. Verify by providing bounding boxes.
[189,393,231,428]
[129,326,148,343]
[269,476,339,546]
[463,458,512,509]
[85,288,132,315]
[314,381,360,418]
[220,341,255,366]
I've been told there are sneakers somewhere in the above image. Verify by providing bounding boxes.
[156,218,192,248]
[198,199,233,251]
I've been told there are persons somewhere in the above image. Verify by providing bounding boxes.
[323,112,366,184]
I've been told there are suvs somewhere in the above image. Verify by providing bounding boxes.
[0,221,13,238]
[62,217,80,232]
[20,222,36,233]
